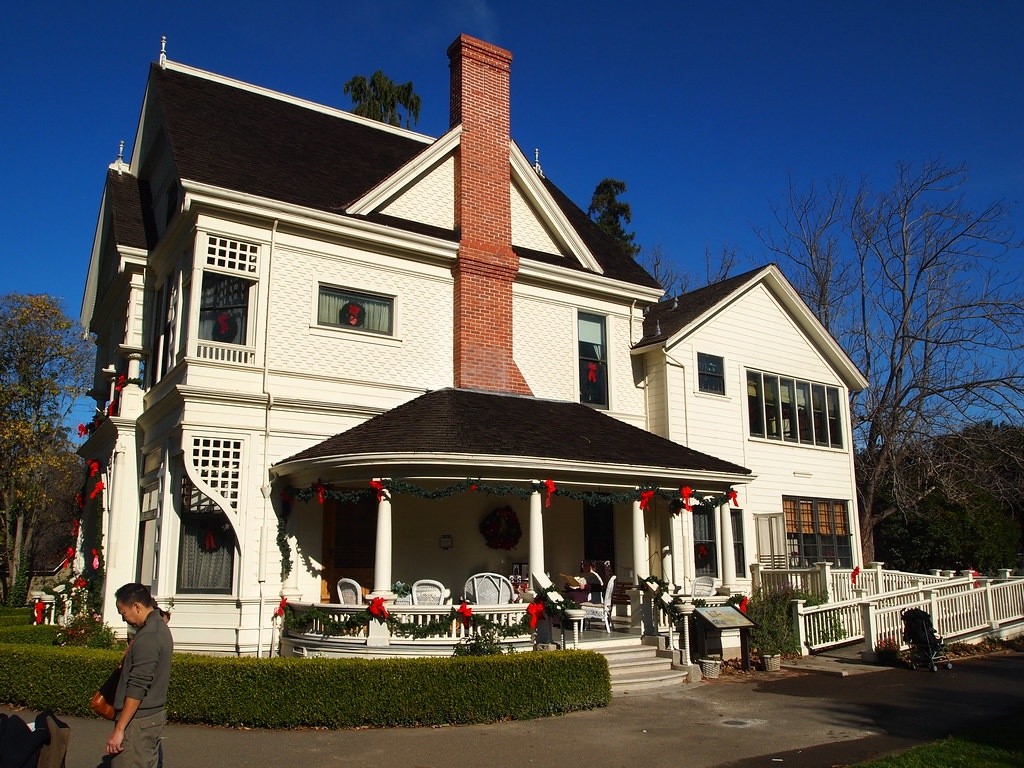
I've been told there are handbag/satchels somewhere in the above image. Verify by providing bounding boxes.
[89,668,122,721]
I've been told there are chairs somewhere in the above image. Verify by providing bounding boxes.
[412,579,446,605]
[464,572,515,605]
[336,578,362,605]
[579,575,618,634]
[691,576,716,597]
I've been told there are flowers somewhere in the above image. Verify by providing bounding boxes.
[874,636,899,652]
[479,504,523,551]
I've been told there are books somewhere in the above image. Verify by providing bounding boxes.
[559,573,579,587]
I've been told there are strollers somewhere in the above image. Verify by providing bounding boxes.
[900,607,953,673]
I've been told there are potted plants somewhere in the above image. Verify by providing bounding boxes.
[699,656,724,680]
[763,647,781,672]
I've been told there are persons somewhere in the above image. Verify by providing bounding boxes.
[32,598,44,626]
[558,561,612,604]
[90,584,173,767]
[73,573,85,595]
